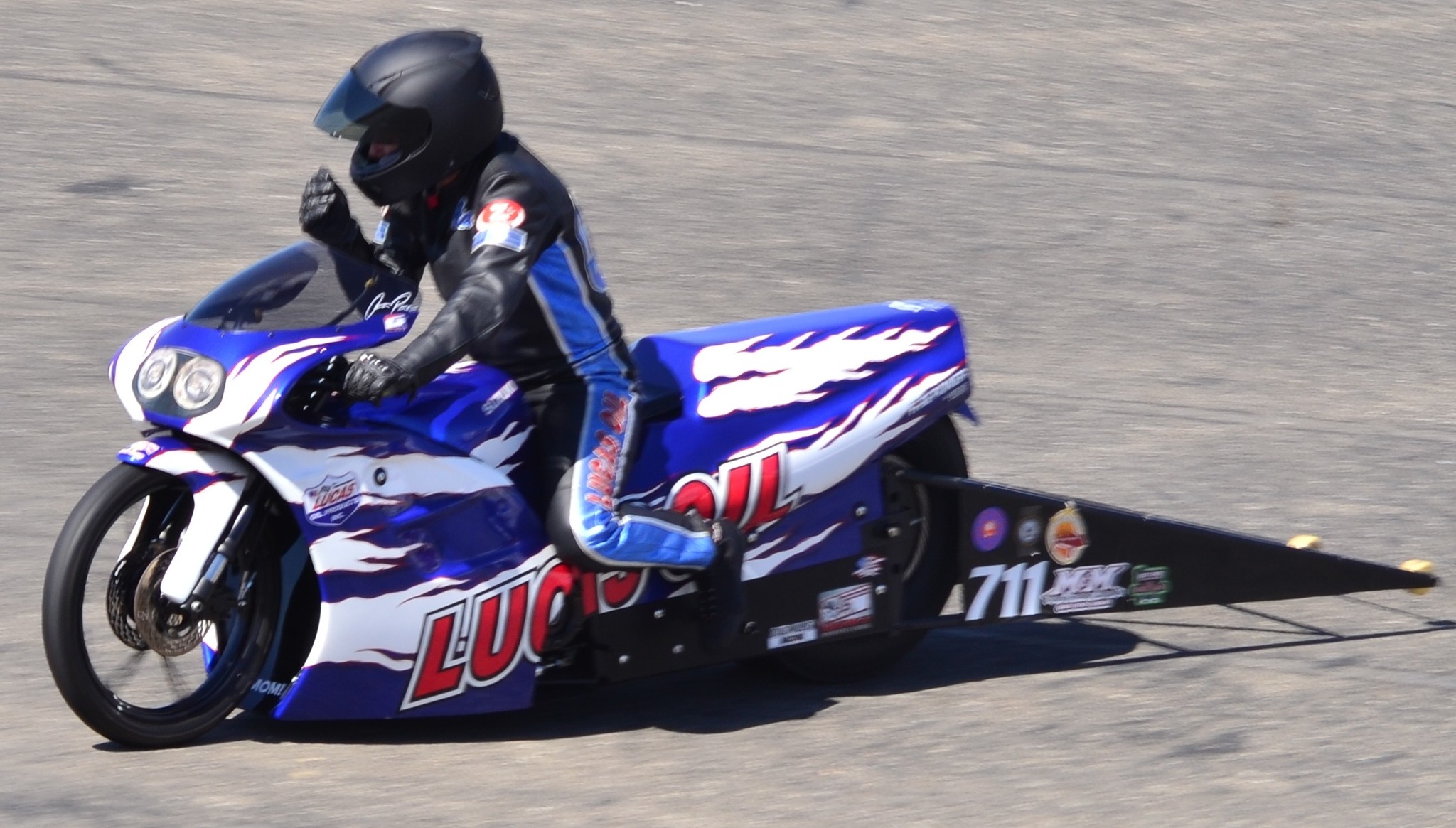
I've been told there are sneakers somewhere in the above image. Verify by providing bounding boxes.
[692,518,745,660]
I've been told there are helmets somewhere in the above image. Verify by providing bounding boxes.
[349,31,504,207]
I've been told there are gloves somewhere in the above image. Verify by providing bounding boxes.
[300,167,351,248]
[343,351,410,408]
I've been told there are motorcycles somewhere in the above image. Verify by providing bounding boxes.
[40,239,983,747]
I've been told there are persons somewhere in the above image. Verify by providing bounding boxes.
[271,26,747,634]
